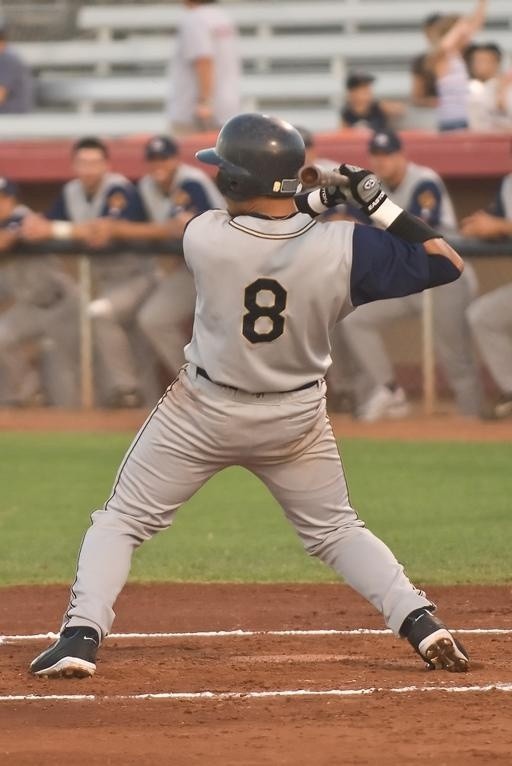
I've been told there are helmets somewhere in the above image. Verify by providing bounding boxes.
[195,113,305,197]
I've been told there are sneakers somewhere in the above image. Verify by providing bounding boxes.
[479,393,511,420]
[399,610,468,673]
[29,626,99,678]
[360,386,409,421]
[99,387,145,407]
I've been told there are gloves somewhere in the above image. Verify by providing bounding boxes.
[320,163,381,206]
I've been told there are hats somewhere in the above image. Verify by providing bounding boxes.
[145,137,175,160]
[369,129,400,153]
[347,70,373,89]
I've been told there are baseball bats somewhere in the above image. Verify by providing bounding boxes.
[299,164,350,188]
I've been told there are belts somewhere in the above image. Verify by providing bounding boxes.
[197,367,318,392]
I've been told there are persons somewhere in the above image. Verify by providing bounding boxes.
[29,114,469,676]
[294,128,511,420]
[1,27,38,115]
[167,1,257,132]
[0,135,225,410]
[340,1,511,134]
